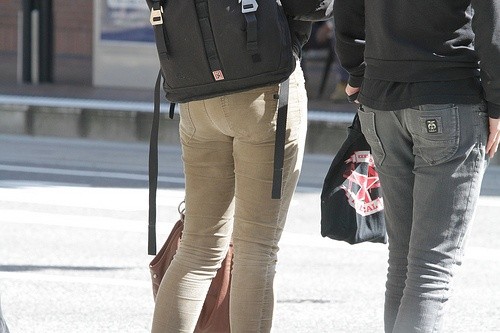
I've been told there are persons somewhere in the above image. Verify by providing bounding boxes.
[332,0,500,333]
[146,0,335,333]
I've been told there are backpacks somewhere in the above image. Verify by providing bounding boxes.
[145,0,297,106]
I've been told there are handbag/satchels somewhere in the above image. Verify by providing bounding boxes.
[148,200,234,333]
[322,88,388,244]
